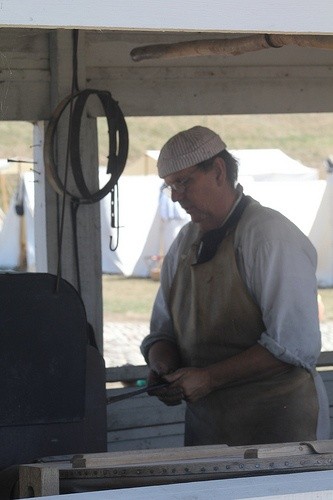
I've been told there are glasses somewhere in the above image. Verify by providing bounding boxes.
[164,163,201,192]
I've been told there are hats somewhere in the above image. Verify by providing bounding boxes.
[155,126,228,178]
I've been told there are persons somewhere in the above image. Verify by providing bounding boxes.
[139,126,322,447]
